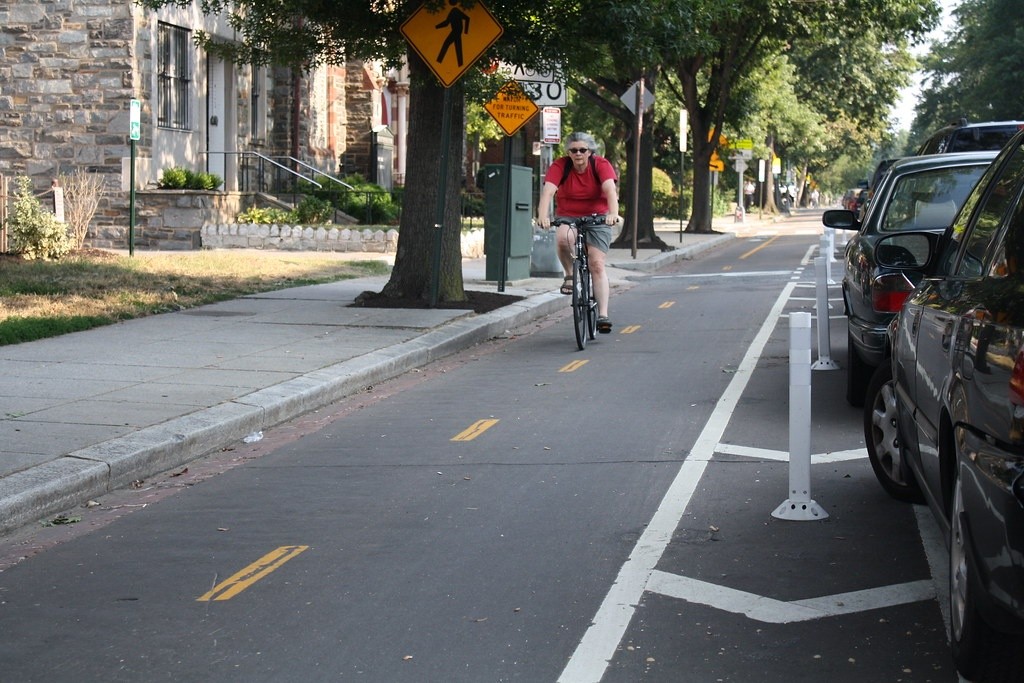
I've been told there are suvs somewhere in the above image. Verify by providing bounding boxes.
[842,118,1024,228]
[862,127,1024,683]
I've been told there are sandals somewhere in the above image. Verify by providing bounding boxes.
[560,275,574,295]
[596,315,612,334]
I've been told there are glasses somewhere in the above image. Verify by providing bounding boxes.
[568,148,588,153]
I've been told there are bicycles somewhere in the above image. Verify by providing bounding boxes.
[539,212,620,350]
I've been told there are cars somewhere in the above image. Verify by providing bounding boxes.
[821,150,1001,408]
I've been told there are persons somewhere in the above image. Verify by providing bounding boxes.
[744,180,755,213]
[538,132,619,333]
[811,189,819,210]
